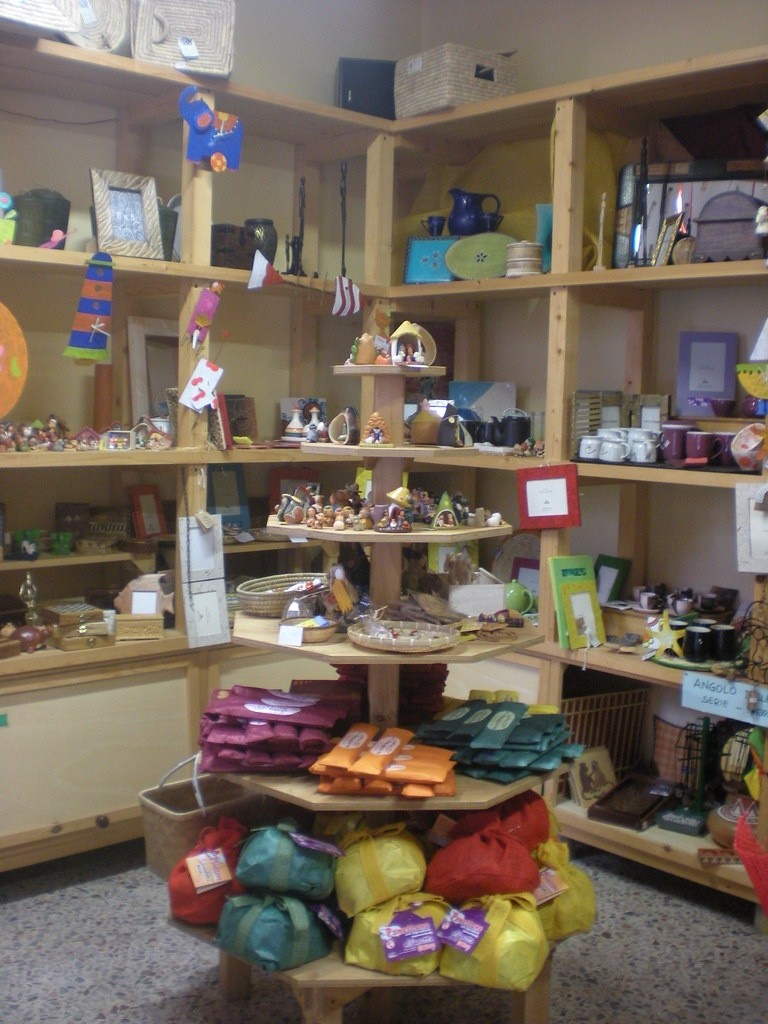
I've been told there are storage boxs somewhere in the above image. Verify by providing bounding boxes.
[41,603,104,626]
[334,56,399,121]
[47,621,108,638]
[114,615,165,640]
[210,223,249,270]
[280,397,327,435]
[55,501,91,532]
[449,379,517,422]
[43,634,115,651]
[587,774,683,833]
[692,191,768,262]
[599,607,664,645]
[0,636,21,661]
[403,235,462,285]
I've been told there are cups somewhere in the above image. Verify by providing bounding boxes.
[578,425,735,463]
[506,240,544,277]
[633,584,737,662]
[421,215,446,236]
[151,419,172,435]
[476,212,504,232]
[458,420,492,445]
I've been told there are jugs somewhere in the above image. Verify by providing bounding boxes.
[448,188,500,234]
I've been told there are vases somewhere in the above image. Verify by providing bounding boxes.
[245,217,278,270]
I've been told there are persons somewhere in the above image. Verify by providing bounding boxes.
[0,419,161,452]
[20,572,37,619]
[305,342,456,530]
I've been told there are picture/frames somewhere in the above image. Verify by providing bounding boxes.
[590,389,627,437]
[428,539,479,580]
[548,553,597,650]
[510,557,540,597]
[593,554,633,602]
[516,464,581,530]
[628,394,671,430]
[207,463,250,532]
[113,574,175,615]
[355,466,409,499]
[90,167,166,260]
[106,430,136,452]
[674,330,739,418]
[650,212,685,265]
[388,320,437,369]
[269,466,319,516]
[734,482,768,575]
[560,580,607,651]
[130,484,167,538]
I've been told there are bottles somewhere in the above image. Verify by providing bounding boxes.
[243,216,278,270]
[284,410,303,437]
[706,794,758,847]
[742,394,768,418]
[302,409,321,438]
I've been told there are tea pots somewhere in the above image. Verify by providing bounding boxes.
[491,408,531,447]
[503,579,533,616]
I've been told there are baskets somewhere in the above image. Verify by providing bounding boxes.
[236,572,329,618]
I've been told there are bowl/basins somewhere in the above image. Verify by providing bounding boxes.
[711,399,736,417]
[730,422,767,452]
[733,451,763,471]
[279,617,340,642]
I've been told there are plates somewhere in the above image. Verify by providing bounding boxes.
[444,232,516,280]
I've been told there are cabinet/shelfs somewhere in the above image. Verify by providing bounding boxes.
[0,26,381,868]
[209,365,571,1024]
[401,41,768,933]
